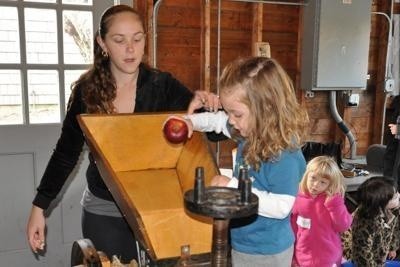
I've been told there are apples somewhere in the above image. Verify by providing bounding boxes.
[163,119,188,144]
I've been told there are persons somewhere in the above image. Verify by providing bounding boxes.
[383,94,400,180]
[290,154,353,267]
[340,176,400,267]
[26,4,222,263]
[161,55,307,267]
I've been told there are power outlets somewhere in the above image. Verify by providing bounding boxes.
[346,93,359,107]
[253,41,271,59]
